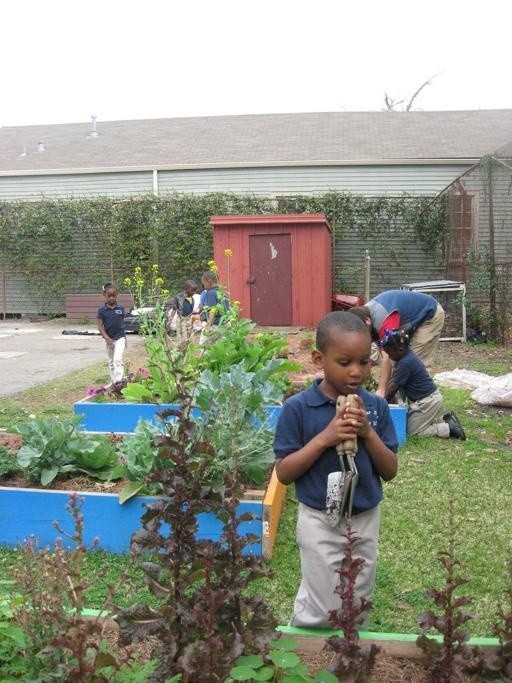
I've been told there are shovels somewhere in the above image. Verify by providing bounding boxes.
[327,394,360,528]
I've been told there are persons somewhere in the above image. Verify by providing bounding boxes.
[191,270,229,360]
[274,311,398,629]
[348,289,466,440]
[166,279,197,342]
[97,283,127,382]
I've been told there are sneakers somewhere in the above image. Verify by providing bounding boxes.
[443,410,466,440]
